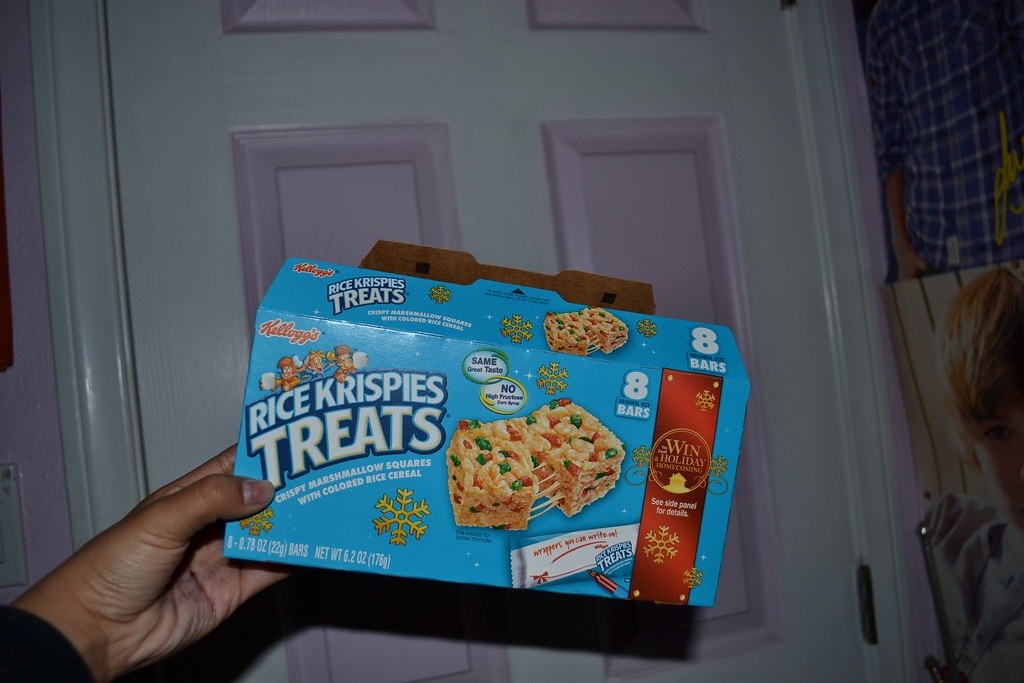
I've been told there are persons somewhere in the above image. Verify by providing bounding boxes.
[0,443,303,683]
[942,267,1024,683]
[859,0,1024,286]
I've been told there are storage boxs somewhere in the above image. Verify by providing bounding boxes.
[222,240,753,611]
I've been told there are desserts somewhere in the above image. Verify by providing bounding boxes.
[543,307,629,357]
[444,398,628,532]
